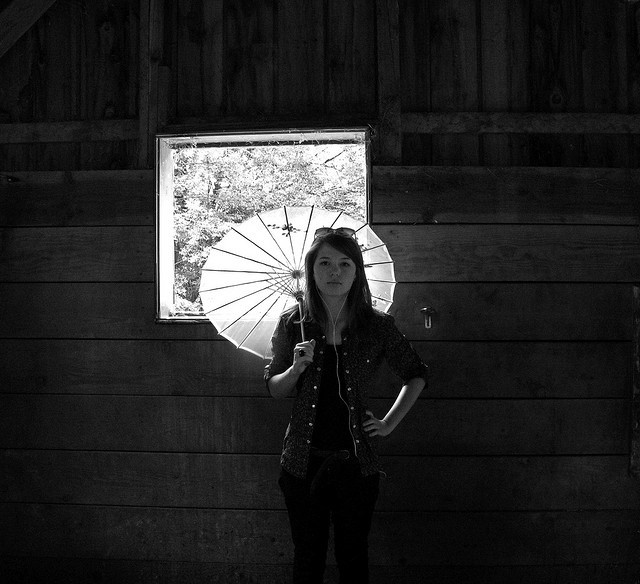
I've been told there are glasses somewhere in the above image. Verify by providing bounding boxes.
[313,227,358,240]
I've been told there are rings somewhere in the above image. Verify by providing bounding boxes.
[297,348,307,358]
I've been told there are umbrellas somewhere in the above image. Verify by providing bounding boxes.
[198,204,398,361]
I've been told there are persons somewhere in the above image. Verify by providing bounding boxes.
[262,226,431,580]
